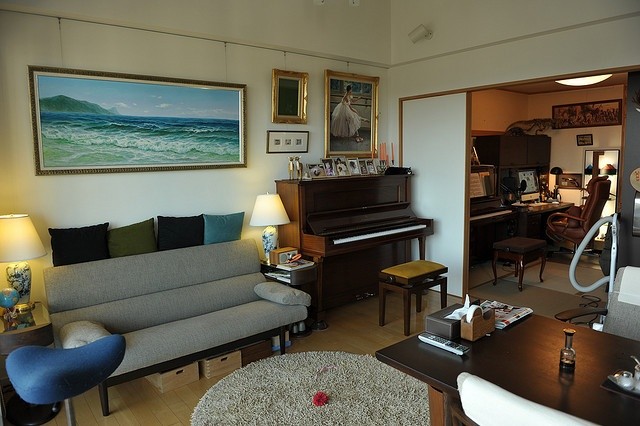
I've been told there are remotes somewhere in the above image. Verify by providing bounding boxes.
[417,330,470,356]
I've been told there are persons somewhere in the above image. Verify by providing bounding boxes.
[336,158,347,176]
[331,85,362,138]
[324,160,333,176]
[350,161,359,174]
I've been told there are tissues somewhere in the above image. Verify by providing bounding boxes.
[425,294,470,340]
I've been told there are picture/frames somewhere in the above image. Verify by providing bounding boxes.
[365,158,377,174]
[271,68,308,124]
[345,157,361,175]
[357,158,370,176]
[27,66,248,176]
[551,99,622,129]
[306,163,327,178]
[331,157,352,177]
[323,68,379,158]
[575,134,593,146]
[320,158,338,176]
[556,173,582,189]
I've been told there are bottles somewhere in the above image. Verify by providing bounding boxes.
[559,329,576,373]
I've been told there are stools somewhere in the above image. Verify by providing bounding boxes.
[492,237,547,292]
[379,259,448,336]
[6,335,127,426]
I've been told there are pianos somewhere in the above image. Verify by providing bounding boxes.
[274,171,434,330]
[469,169,518,269]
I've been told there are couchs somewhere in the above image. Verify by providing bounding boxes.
[43,240,311,418]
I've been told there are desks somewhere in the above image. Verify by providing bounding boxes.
[512,199,574,237]
[470,209,518,266]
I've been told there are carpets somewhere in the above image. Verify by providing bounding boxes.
[190,350,430,426]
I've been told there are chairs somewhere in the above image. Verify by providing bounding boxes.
[554,265,640,343]
[546,176,611,260]
[456,371,599,425]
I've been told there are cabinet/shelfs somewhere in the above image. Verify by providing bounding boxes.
[260,262,316,337]
[477,134,551,197]
[0,303,62,425]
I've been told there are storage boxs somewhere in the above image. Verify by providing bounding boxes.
[144,359,201,393]
[199,350,242,379]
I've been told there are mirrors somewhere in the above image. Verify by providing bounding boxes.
[632,191,640,236]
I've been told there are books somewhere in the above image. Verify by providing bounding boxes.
[276,259,315,272]
[480,300,534,330]
[264,271,291,284]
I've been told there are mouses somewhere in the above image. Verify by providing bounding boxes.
[552,201,560,205]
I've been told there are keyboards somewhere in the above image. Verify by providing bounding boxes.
[527,201,548,207]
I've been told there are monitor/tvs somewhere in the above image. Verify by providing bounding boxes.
[518,169,541,202]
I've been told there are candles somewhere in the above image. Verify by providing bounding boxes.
[383,142,386,160]
[380,143,383,159]
[391,143,394,159]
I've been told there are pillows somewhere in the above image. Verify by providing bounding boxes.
[59,321,112,350]
[253,282,312,308]
[567,206,581,227]
[204,212,245,244]
[108,218,156,258]
[156,215,205,250]
[48,221,109,267]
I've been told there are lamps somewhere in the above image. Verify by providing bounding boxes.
[249,192,291,260]
[408,24,432,44]
[549,166,563,199]
[0,213,47,306]
